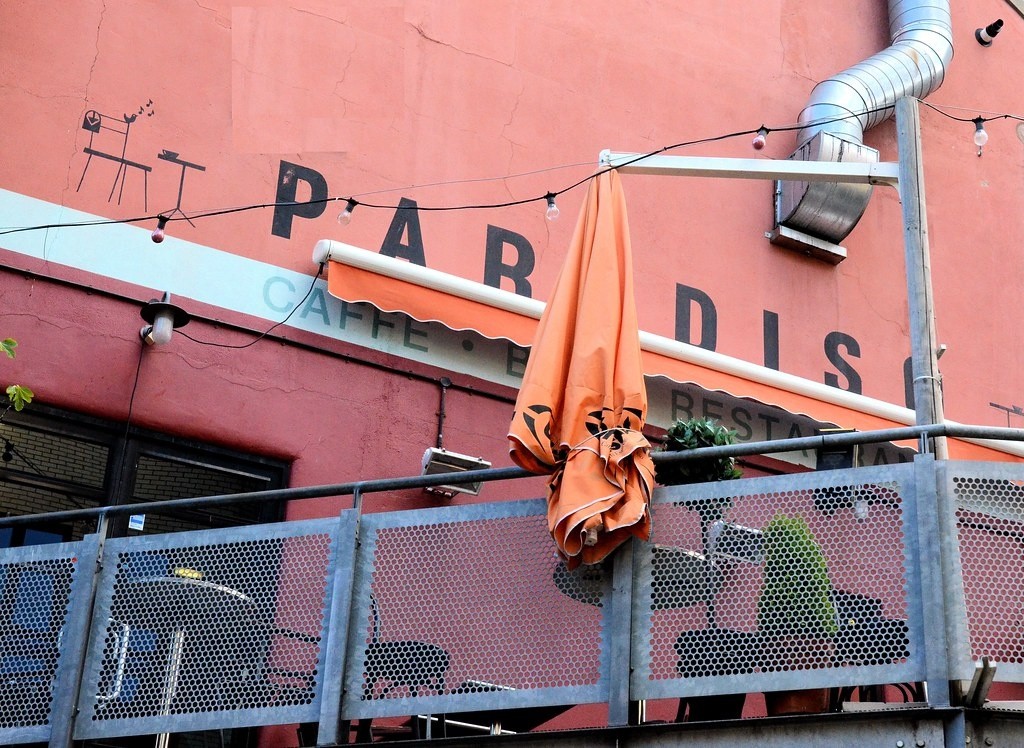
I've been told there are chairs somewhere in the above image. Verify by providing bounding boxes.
[231,592,380,746]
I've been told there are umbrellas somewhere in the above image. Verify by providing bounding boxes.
[506,155,660,567]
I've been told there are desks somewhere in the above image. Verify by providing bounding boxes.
[552,541,722,725]
[105,577,250,748]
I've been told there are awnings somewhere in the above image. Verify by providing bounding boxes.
[312,236,1024,486]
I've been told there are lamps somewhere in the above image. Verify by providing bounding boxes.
[420,446,492,498]
[140,289,190,349]
[707,518,764,565]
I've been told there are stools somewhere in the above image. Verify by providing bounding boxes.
[363,638,447,737]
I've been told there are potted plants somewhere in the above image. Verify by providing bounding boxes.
[755,509,838,712]
[652,417,761,720]
